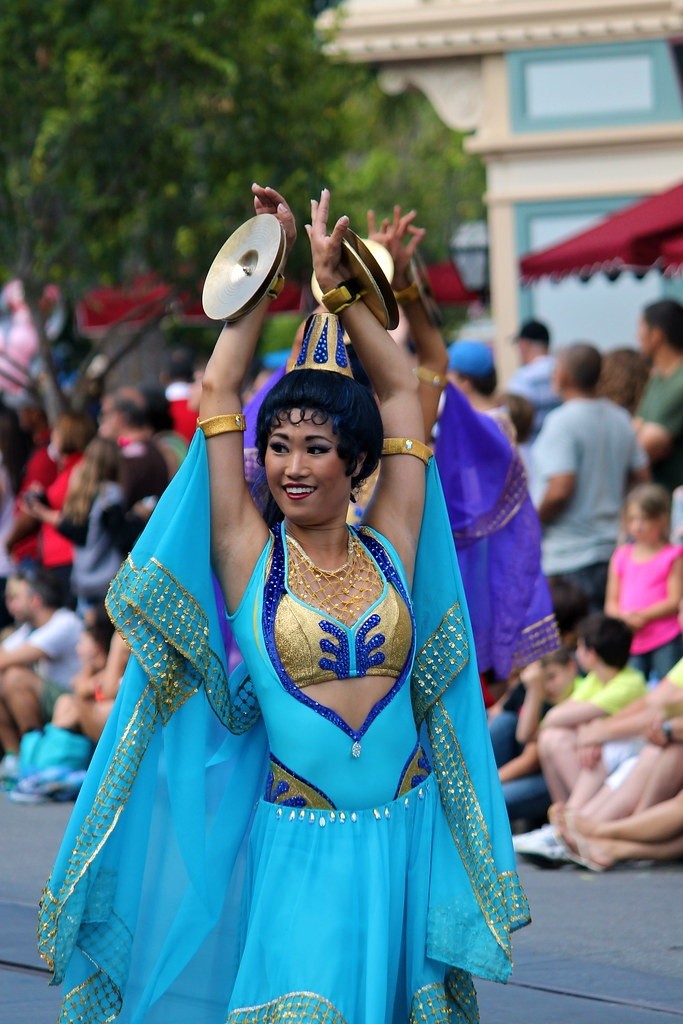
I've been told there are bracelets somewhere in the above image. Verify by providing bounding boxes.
[94,684,103,703]
[663,722,673,741]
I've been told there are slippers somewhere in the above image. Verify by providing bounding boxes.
[547,802,607,872]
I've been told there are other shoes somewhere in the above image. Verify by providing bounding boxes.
[510,820,563,874]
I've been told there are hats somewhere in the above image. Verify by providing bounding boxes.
[443,340,493,376]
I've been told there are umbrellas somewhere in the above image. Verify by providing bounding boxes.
[518,185,683,284]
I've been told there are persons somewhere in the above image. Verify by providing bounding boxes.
[35,180,559,1024]
[0,279,683,871]
